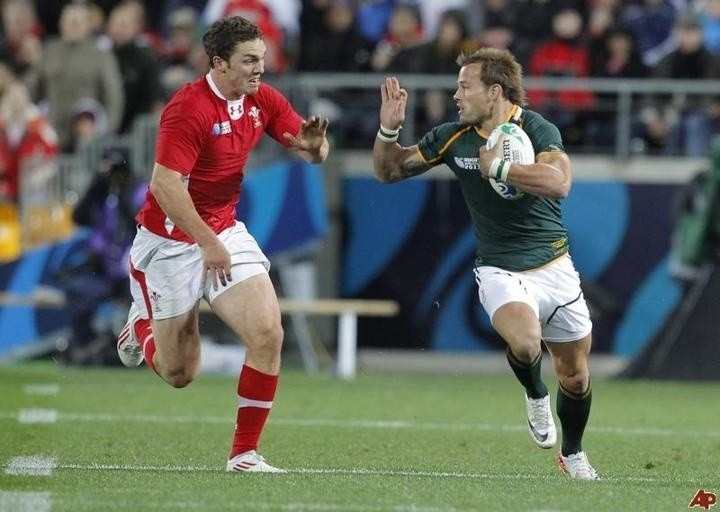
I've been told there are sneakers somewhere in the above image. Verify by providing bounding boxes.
[557,444,601,481]
[225,450,288,474]
[117,302,151,368]
[525,391,557,449]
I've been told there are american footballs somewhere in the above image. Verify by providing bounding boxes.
[486,123,535,200]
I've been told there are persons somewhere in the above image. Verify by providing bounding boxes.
[117,15,328,473]
[371,45,605,479]
[53,144,155,368]
